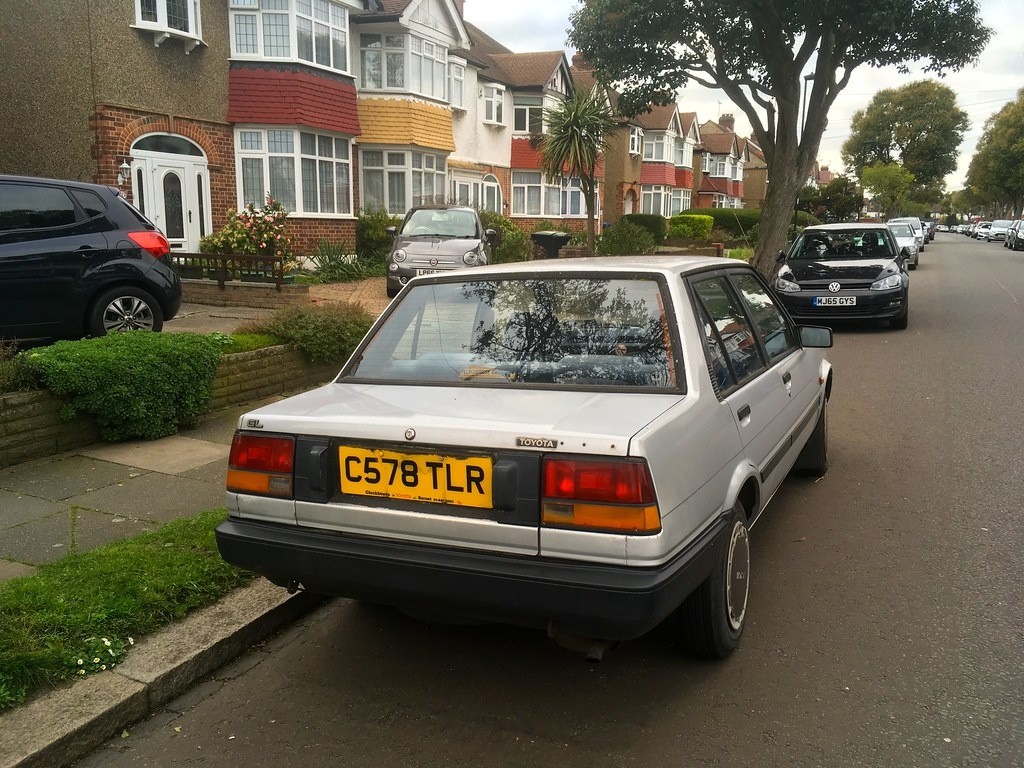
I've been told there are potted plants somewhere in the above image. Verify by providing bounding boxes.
[181,259,202,279]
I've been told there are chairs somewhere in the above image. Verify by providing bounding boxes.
[862,233,879,251]
[503,309,563,360]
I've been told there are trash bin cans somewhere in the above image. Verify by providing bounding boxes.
[530,230,571,259]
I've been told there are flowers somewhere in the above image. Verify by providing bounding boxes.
[200,191,301,272]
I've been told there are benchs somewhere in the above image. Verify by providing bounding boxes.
[394,348,666,388]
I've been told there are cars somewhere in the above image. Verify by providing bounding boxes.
[1004,219,1024,251]
[888,216,991,253]
[767,223,911,330]
[385,205,496,297]
[989,220,1013,242]
[884,223,921,270]
[215,257,836,661]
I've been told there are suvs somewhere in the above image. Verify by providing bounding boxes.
[0,175,183,348]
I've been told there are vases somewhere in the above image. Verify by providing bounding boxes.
[208,269,233,281]
[265,275,293,285]
[241,272,263,282]
